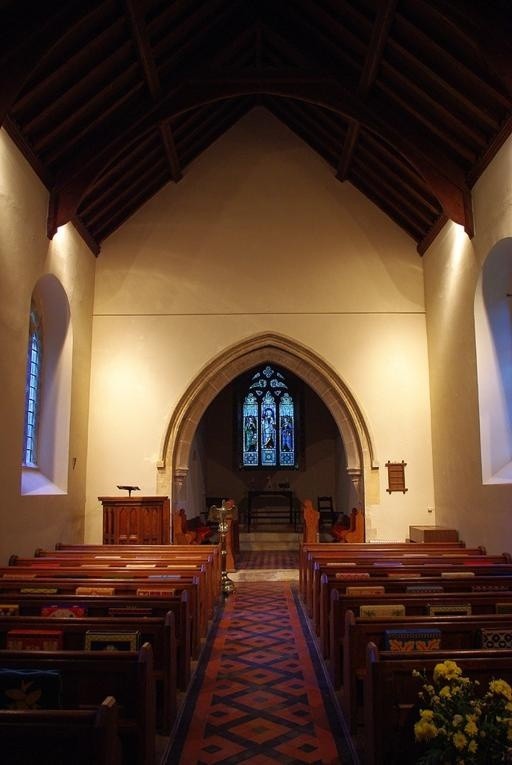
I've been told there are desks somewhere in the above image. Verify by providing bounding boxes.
[246,490,293,533]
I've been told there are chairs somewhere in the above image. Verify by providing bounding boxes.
[338,510,364,543]
[174,508,197,543]
[317,496,334,530]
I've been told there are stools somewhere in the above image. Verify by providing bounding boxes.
[296,523,304,532]
